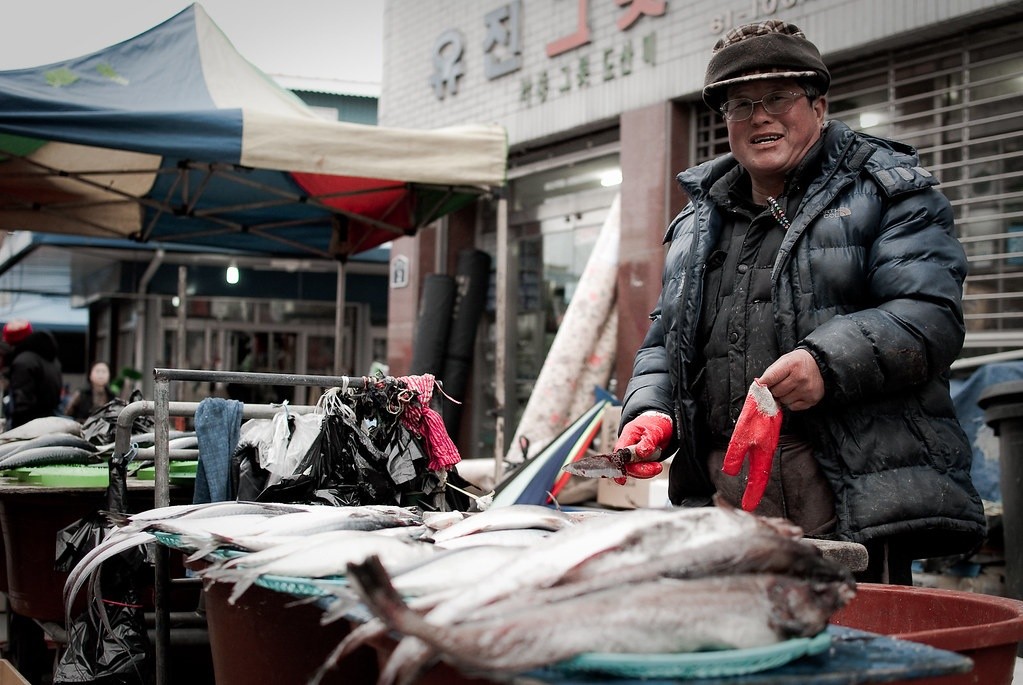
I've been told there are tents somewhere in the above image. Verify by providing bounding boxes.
[0,2,509,502]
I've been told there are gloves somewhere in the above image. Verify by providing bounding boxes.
[721,378,783,513]
[613,410,673,485]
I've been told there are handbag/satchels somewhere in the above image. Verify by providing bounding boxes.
[52,511,154,683]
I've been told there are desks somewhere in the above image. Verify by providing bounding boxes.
[151,527,975,685]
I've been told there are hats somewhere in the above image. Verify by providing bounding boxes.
[702,19,830,114]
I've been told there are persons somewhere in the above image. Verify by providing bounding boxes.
[3,330,119,428]
[612,20,988,589]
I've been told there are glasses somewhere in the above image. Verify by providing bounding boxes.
[718,90,812,122]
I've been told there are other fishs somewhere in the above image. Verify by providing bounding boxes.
[0,416,105,469]
[96,430,199,462]
[65,491,859,685]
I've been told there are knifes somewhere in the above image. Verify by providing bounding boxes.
[562,443,663,478]
